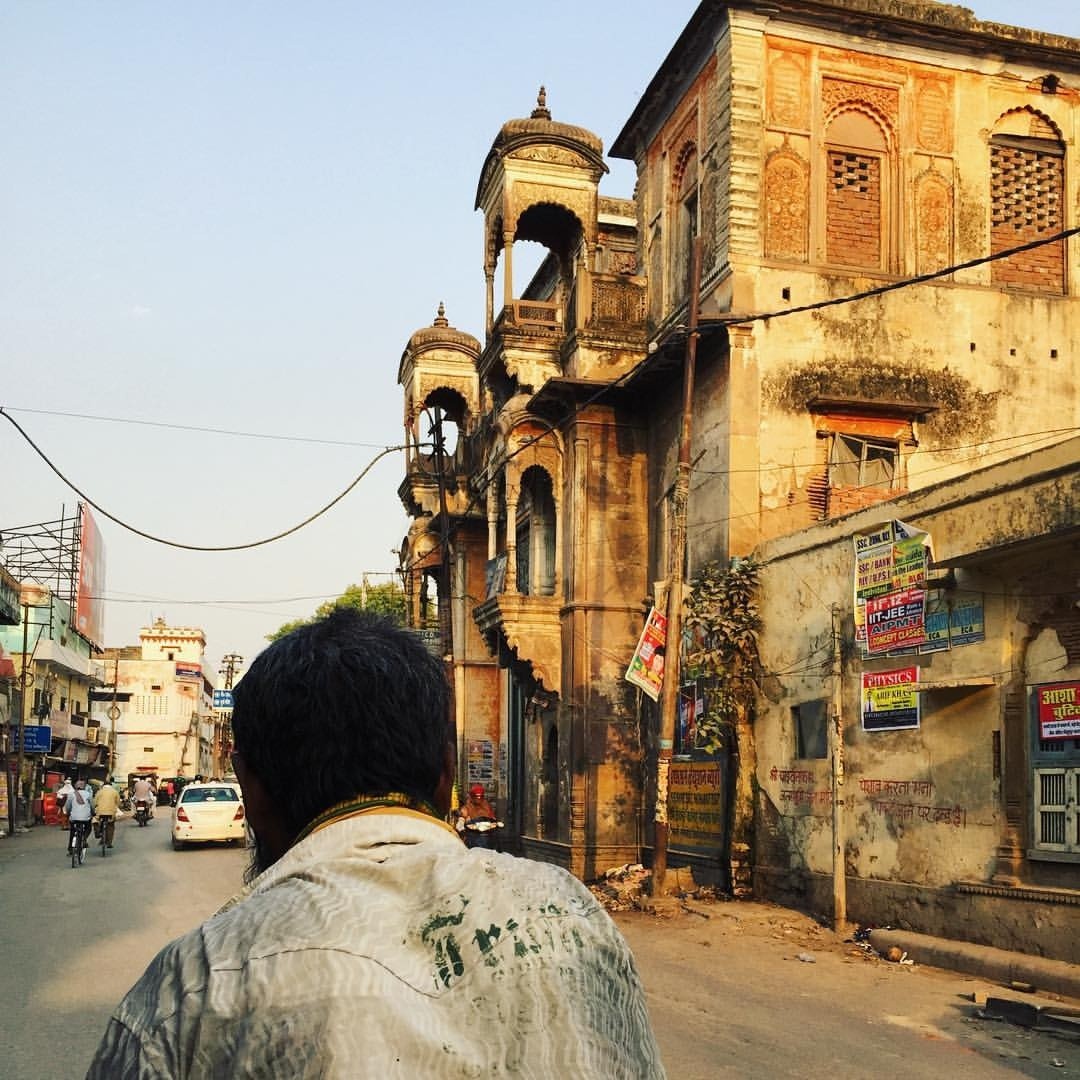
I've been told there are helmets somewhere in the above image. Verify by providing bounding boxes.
[469,784,484,797]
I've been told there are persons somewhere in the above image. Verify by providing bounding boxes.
[109,776,121,794]
[92,780,120,847]
[132,776,153,816]
[455,785,505,839]
[60,775,94,855]
[83,606,668,1080]
[168,768,219,806]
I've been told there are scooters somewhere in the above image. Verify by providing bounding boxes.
[453,811,505,850]
[132,799,154,826]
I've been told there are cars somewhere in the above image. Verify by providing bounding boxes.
[170,782,250,849]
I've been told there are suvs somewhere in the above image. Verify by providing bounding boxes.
[157,777,195,804]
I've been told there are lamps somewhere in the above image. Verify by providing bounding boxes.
[1026,74,1059,93]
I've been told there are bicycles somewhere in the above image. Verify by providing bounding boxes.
[98,814,109,857]
[69,822,90,868]
